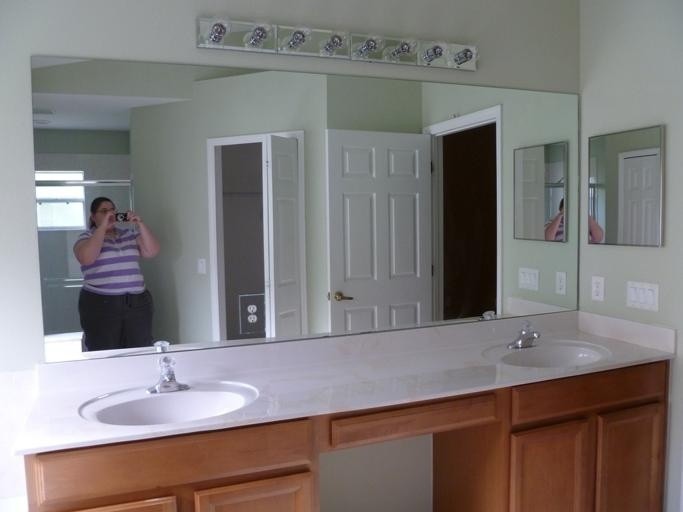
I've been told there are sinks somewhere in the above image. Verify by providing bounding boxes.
[79,382,260,426]
[108,346,200,357]
[480,341,613,368]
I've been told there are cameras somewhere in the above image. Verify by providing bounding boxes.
[115,213,129,222]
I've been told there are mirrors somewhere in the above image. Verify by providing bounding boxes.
[27,50,583,366]
[511,141,569,244]
[587,123,666,249]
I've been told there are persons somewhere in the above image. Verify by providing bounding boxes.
[544,197,604,244]
[73,196,160,351]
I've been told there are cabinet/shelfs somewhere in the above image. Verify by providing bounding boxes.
[429,359,667,512]
[27,419,315,512]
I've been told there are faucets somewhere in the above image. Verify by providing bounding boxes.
[508,320,541,348]
[154,340,170,354]
[147,357,190,394]
[479,311,496,321]
